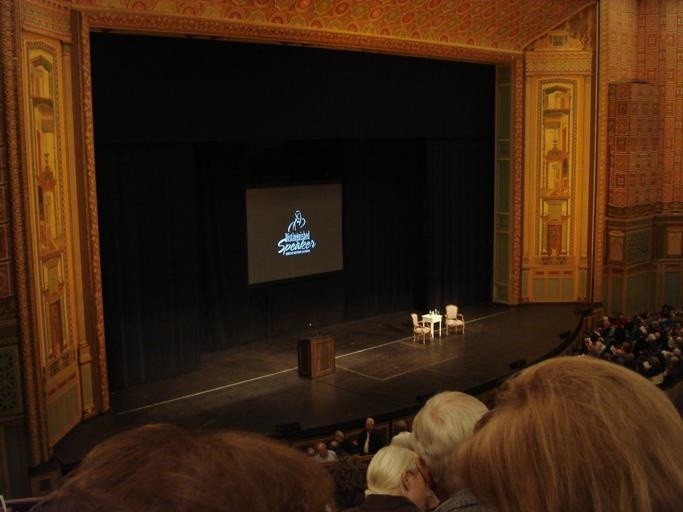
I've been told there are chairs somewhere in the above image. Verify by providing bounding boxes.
[443,304,465,336]
[410,313,432,345]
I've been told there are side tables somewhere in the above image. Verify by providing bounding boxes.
[420,313,443,340]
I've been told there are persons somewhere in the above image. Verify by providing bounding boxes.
[305,417,412,463]
[349,355,683,511]
[23,421,339,510]
[579,305,683,391]
